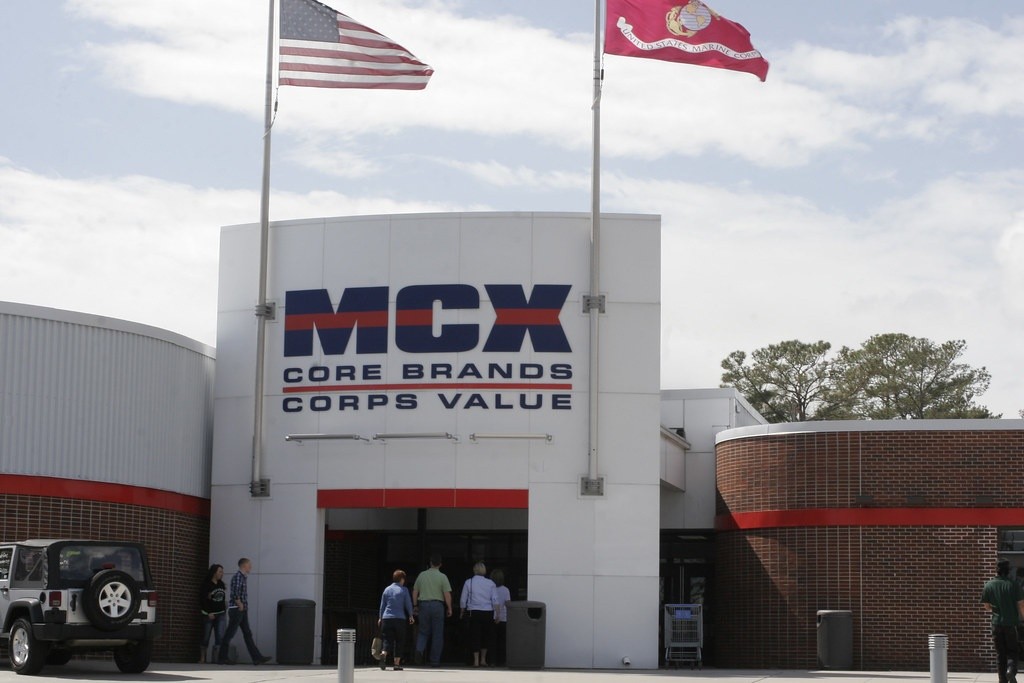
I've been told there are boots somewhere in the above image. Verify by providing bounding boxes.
[213,645,220,663]
[197,645,207,663]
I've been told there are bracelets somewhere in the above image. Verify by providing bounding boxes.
[414,605,418,607]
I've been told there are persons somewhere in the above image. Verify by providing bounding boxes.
[412,552,452,666]
[378,570,414,670]
[459,562,510,666]
[982,559,1024,683]
[200,558,272,665]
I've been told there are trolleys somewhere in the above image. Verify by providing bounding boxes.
[664,603,704,671]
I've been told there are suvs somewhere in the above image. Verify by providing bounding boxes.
[0,538,158,675]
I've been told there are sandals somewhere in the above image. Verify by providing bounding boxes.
[379,654,387,670]
[394,664,403,670]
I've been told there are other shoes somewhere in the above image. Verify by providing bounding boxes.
[1006,670,1017,683]
[414,650,423,664]
[472,664,479,667]
[999,675,1006,683]
[480,662,488,667]
[253,656,271,665]
[430,664,440,667]
[217,659,236,665]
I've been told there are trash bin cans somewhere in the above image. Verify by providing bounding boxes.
[504,600,547,671]
[275,597,316,666]
[816,610,854,670]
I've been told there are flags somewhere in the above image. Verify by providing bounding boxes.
[266,0,434,91]
[602,0,769,83]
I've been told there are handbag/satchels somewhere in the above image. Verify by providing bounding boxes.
[457,608,472,619]
[370,623,382,660]
